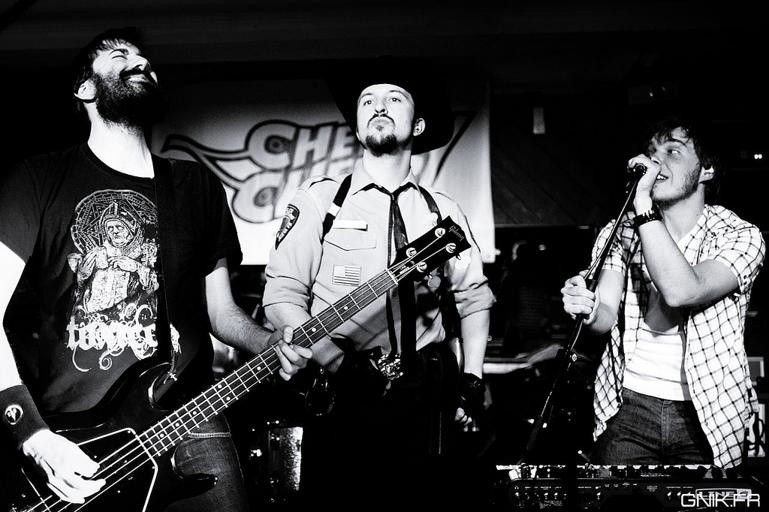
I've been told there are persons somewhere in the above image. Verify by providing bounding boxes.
[0,15,315,505]
[562,112,767,467]
[262,58,499,509]
[489,220,598,350]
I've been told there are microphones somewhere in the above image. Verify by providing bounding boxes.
[628,163,647,177]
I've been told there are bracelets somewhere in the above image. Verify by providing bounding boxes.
[0,382,50,456]
[631,208,664,236]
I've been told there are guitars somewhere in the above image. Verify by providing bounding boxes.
[1,216,471,511]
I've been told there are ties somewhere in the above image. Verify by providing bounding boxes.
[368,184,416,347]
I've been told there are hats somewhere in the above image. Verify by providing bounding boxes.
[333,53,455,155]
